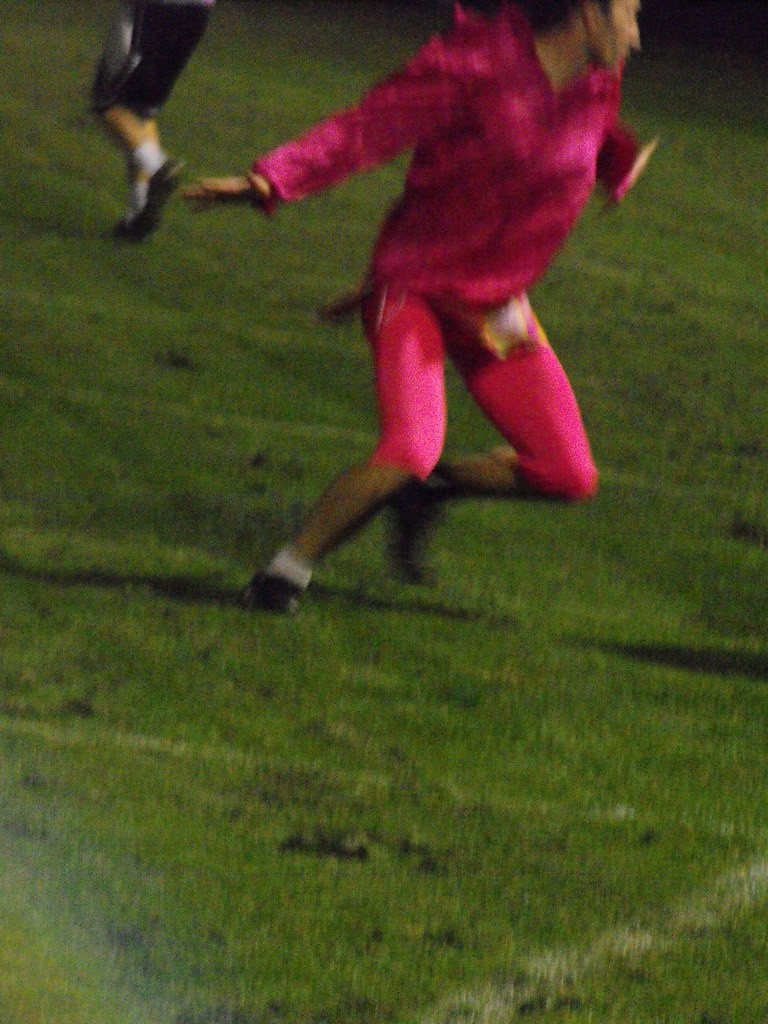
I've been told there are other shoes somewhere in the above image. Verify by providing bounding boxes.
[391,476,438,580]
[113,160,183,241]
[250,573,307,616]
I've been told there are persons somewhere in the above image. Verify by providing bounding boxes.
[173,1,665,619]
[81,1,214,246]
